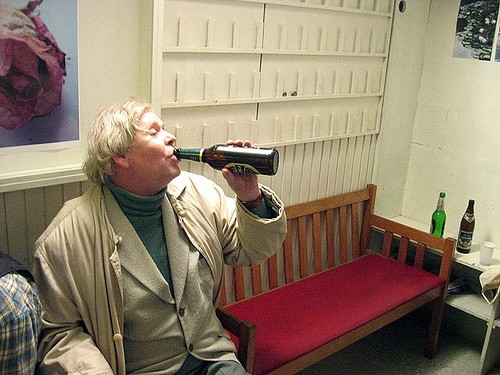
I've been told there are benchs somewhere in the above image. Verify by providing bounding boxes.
[213,183,458,375]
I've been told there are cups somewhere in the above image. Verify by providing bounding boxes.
[479,241,496,266]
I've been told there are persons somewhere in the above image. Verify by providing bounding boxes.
[0,251,43,375]
[31,96,288,375]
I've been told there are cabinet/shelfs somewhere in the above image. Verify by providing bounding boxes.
[151,0,398,149]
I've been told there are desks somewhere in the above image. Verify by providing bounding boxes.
[370,214,500,375]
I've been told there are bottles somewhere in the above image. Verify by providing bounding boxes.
[173,143,280,176]
[430,192,446,238]
[456,199,475,254]
